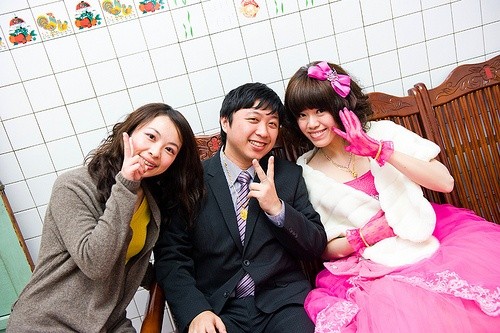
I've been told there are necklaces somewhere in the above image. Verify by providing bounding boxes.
[318,146,359,178]
[221,152,257,220]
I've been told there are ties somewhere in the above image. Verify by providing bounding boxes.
[235,171,254,298]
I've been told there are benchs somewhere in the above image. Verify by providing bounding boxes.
[141,53,500,333]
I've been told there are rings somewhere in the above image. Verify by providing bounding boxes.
[262,177,268,183]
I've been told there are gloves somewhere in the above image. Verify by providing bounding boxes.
[347,208,397,259]
[331,106,394,167]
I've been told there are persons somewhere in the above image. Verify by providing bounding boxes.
[6,102,208,333]
[285,61,500,333]
[152,82,327,333]
